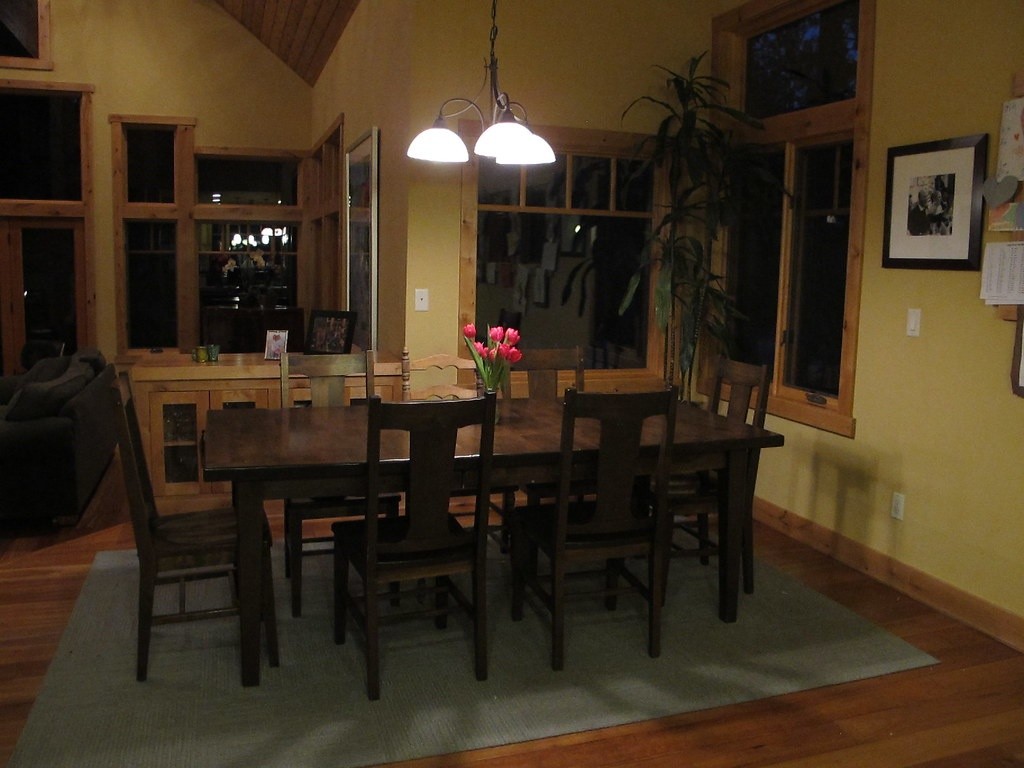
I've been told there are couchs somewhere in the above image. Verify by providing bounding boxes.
[0,349,120,538]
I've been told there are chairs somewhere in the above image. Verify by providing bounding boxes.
[21,340,65,370]
[110,345,772,702]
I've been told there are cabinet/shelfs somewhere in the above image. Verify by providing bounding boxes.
[131,344,403,497]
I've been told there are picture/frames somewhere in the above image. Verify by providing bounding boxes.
[558,212,587,258]
[264,330,288,360]
[303,310,358,355]
[881,132,989,270]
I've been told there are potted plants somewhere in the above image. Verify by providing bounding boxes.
[622,46,795,500]
[9,514,942,768]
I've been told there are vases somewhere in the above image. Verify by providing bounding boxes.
[484,387,500,425]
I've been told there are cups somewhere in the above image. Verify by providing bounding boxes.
[195,346,207,362]
[207,344,220,360]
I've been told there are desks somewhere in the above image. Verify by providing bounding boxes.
[204,397,784,686]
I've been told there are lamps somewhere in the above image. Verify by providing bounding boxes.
[407,0,557,165]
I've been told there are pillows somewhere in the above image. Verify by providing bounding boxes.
[14,356,72,394]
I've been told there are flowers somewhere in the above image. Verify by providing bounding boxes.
[462,325,523,389]
